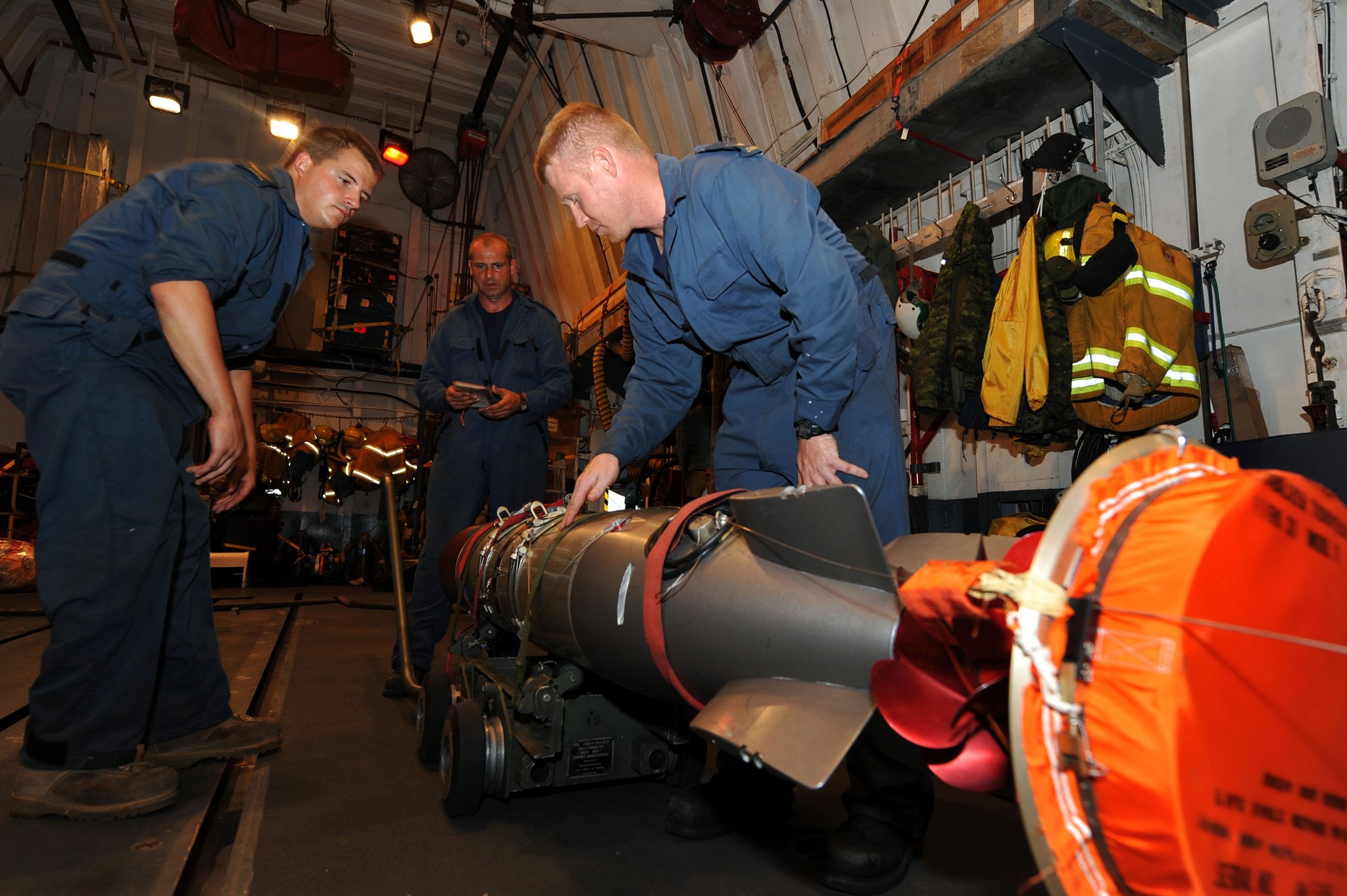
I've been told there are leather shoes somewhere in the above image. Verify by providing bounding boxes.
[659,746,801,840]
[140,710,285,771]
[11,760,185,823]
[382,668,409,698]
[809,779,931,896]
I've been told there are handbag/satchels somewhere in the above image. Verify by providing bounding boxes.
[1072,198,1139,297]
[1018,132,1085,223]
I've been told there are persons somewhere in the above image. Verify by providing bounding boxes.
[533,100,938,894]
[381,233,575,700]
[2,119,388,822]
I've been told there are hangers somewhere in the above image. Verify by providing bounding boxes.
[256,403,416,439]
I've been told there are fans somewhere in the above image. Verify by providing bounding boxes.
[398,147,486,231]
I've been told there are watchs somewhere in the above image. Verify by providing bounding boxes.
[794,419,839,440]
[517,393,527,413]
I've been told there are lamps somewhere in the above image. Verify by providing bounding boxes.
[143,74,190,115]
[407,0,434,45]
[378,128,410,166]
[265,104,306,142]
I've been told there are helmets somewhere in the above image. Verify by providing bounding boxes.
[342,426,367,448]
[895,289,931,341]
[259,422,287,444]
[309,425,334,445]
[403,436,420,459]
[1042,224,1075,284]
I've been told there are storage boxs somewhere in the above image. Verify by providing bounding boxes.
[0,122,129,307]
[782,136,817,171]
[819,0,1014,149]
[573,270,628,336]
[311,223,415,361]
